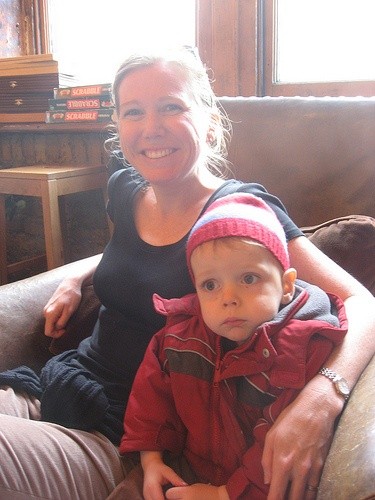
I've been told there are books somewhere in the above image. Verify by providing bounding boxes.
[46,83,112,124]
[0,53,76,123]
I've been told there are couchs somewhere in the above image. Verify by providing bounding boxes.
[0,97,375,500]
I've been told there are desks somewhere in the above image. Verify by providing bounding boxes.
[0,122,123,176]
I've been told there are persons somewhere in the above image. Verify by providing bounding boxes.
[0,49,375,500]
[121,194,349,500]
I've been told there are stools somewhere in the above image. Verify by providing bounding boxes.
[0,163,115,286]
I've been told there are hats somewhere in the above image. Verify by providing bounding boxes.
[186,192,290,288]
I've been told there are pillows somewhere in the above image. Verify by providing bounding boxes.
[298,215,375,297]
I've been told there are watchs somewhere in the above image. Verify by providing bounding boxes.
[318,368,350,401]
